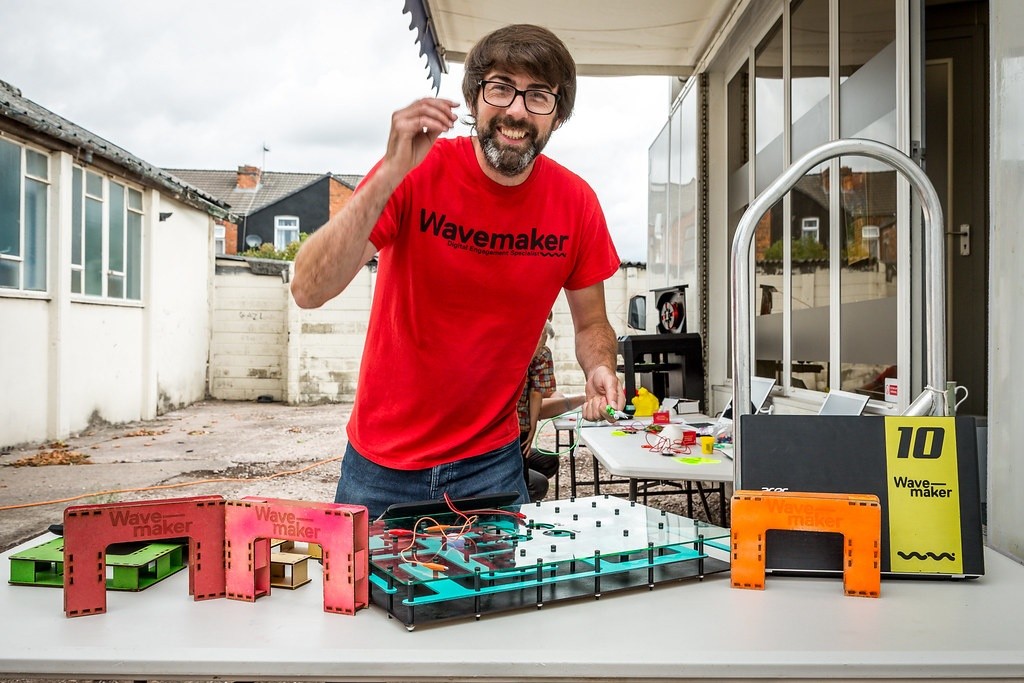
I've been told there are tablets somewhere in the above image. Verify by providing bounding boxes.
[376,492,521,531]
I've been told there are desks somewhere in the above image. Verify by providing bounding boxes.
[0,524,1022,683]
[614,332,703,413]
[552,409,735,528]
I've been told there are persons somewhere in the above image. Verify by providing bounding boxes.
[290,24,627,523]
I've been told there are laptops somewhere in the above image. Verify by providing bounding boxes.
[680,377,777,435]
[719,389,871,461]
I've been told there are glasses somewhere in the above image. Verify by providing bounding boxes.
[478,80,560,115]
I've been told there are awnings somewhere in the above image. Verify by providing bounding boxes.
[402,1,739,97]
